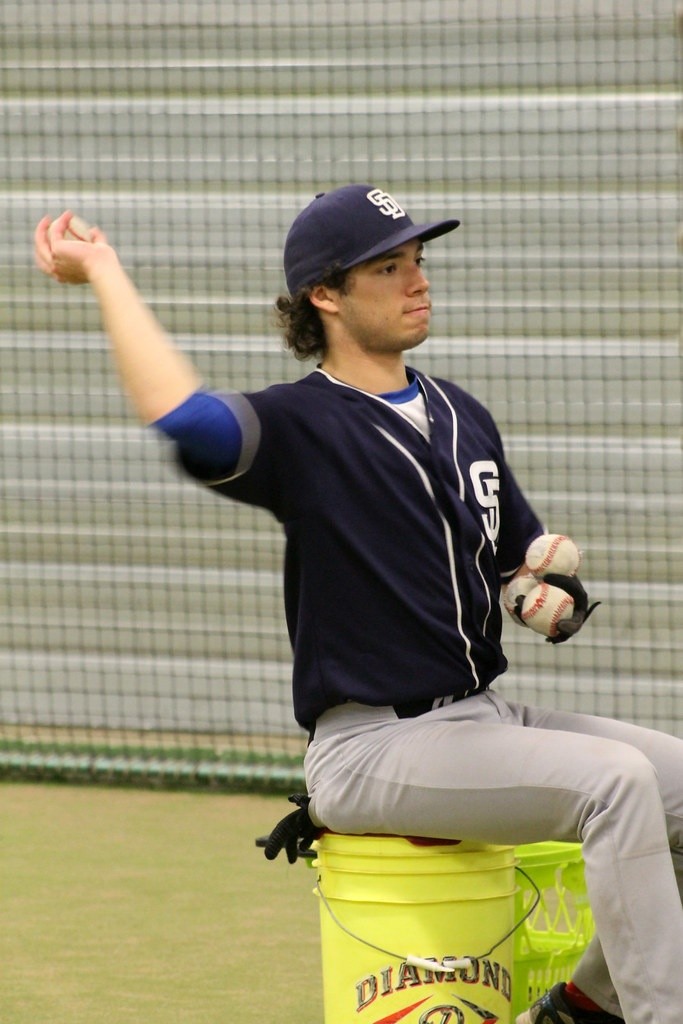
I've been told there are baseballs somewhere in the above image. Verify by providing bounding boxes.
[522,532,578,576]
[500,571,540,615]
[40,211,93,244]
[519,584,577,632]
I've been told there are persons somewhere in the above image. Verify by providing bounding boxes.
[34,185,683,1024]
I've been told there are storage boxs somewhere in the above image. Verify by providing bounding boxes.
[510,841,595,1019]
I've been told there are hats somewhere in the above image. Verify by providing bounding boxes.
[283,184,461,296]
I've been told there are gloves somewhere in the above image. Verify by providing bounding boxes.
[513,573,602,645]
[265,793,323,864]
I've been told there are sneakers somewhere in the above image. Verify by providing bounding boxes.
[515,981,575,1024]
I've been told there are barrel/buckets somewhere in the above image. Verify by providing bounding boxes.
[308,831,540,1024]
